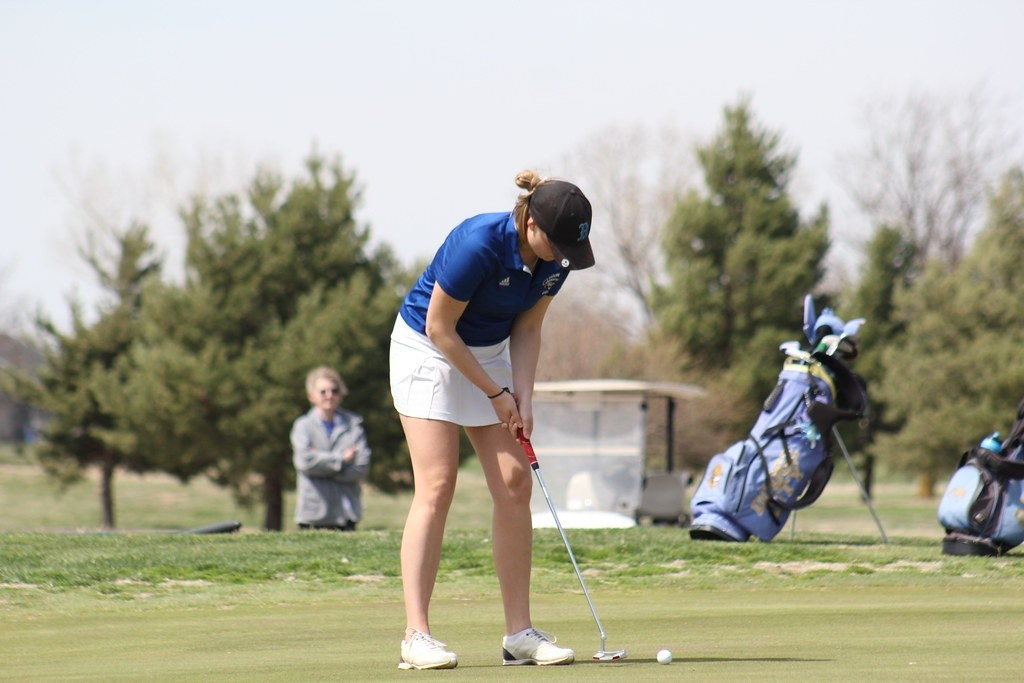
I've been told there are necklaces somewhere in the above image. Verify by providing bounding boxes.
[488,389,506,399]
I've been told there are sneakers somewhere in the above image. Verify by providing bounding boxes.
[398,632,459,670]
[502,628,575,666]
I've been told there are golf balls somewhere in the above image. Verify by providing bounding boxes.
[657,650,672,664]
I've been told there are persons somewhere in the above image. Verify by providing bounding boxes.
[290,365,370,532]
[389,169,596,669]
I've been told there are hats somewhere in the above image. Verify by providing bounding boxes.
[528,180,595,269]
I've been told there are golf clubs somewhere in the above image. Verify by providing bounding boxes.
[503,387,627,661]
[779,294,866,366]
